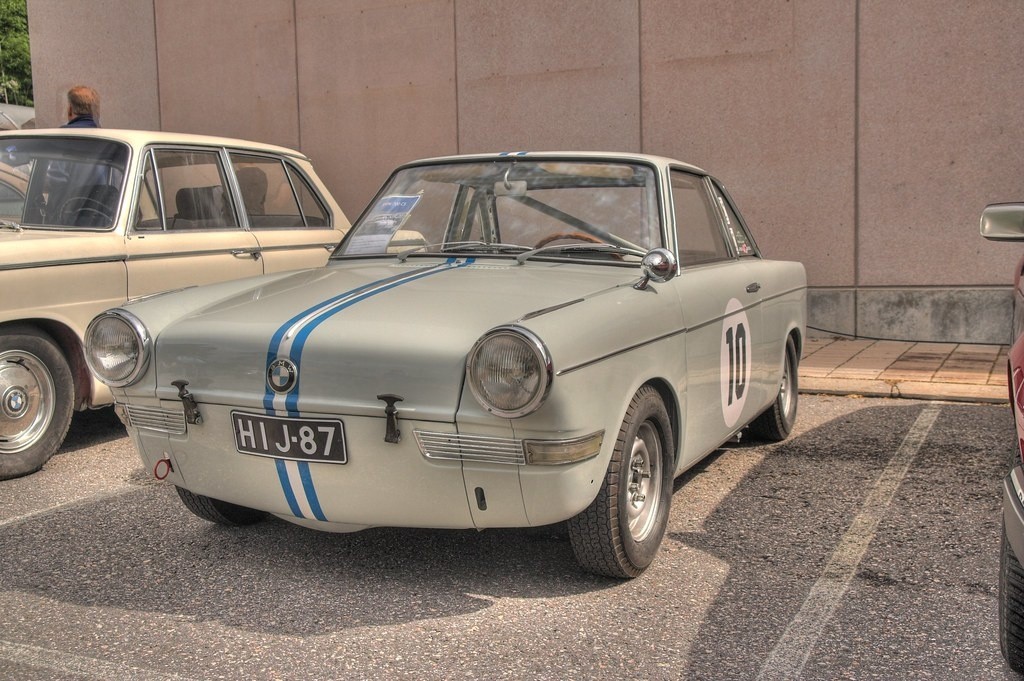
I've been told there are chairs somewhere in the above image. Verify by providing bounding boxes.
[62,185,233,229]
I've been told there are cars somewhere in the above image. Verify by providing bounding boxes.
[0,127,429,482]
[975,200,1024,672]
[0,158,52,232]
[82,147,808,582]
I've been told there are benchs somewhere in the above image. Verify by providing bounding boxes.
[139,215,325,230]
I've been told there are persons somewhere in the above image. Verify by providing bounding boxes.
[44,86,144,224]
